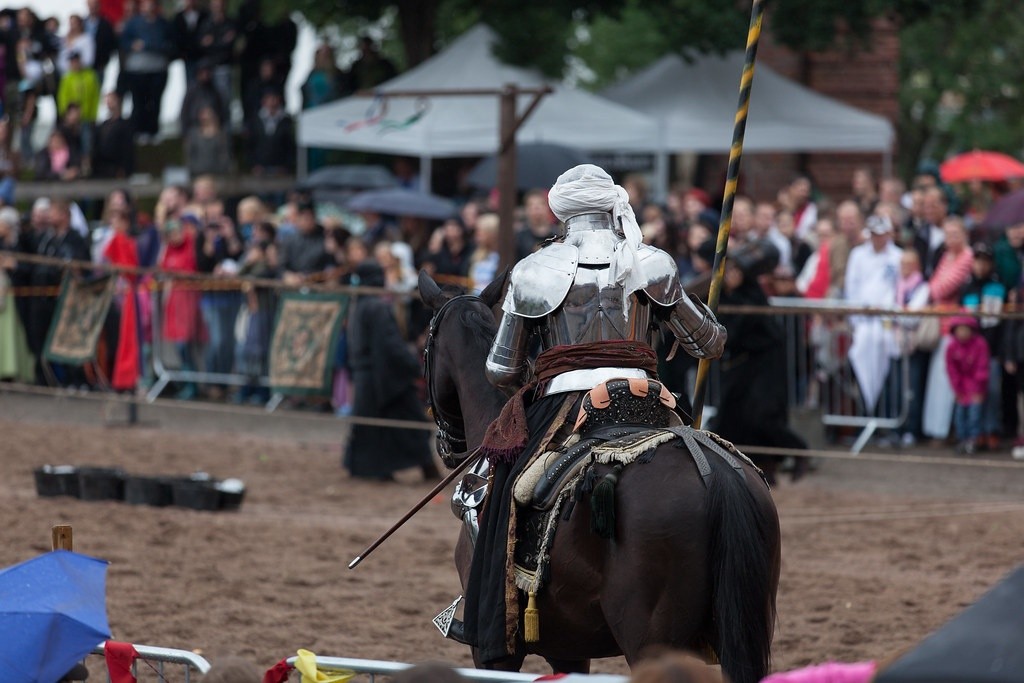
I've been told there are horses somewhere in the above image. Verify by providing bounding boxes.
[418,264,782,683]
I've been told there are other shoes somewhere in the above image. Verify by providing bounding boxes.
[821,422,1023,461]
[0,368,354,415]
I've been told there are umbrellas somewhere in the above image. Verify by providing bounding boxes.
[1,550,115,683]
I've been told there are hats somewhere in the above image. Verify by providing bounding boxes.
[548,162,619,219]
[865,211,894,235]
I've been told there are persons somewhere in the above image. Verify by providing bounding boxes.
[0,0,1024,486]
[447,163,726,645]
[55,663,88,683]
[202,651,740,683]
